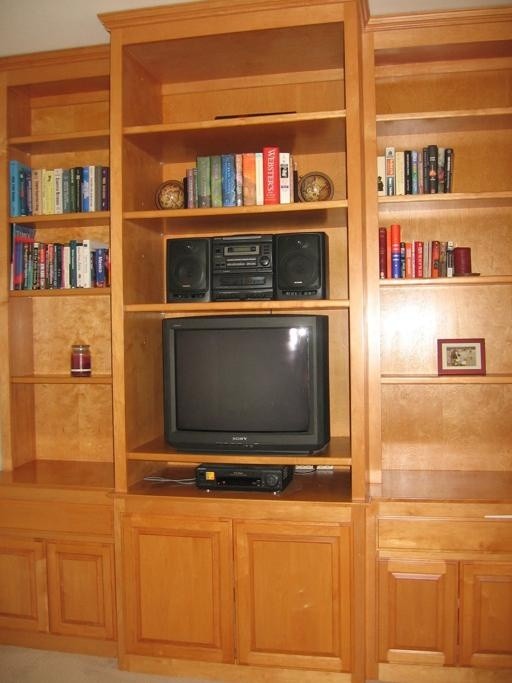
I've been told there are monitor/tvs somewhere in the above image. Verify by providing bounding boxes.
[158,315,331,455]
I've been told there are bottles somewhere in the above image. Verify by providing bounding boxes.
[70,343,92,377]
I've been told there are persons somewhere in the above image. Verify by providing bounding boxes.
[451,347,474,366]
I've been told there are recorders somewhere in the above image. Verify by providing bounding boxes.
[164,232,331,301]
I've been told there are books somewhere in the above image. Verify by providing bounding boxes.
[376,146,471,279]
[184,144,298,208]
[7,157,111,290]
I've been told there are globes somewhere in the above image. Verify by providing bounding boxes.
[155,180,184,209]
[298,171,334,202]
